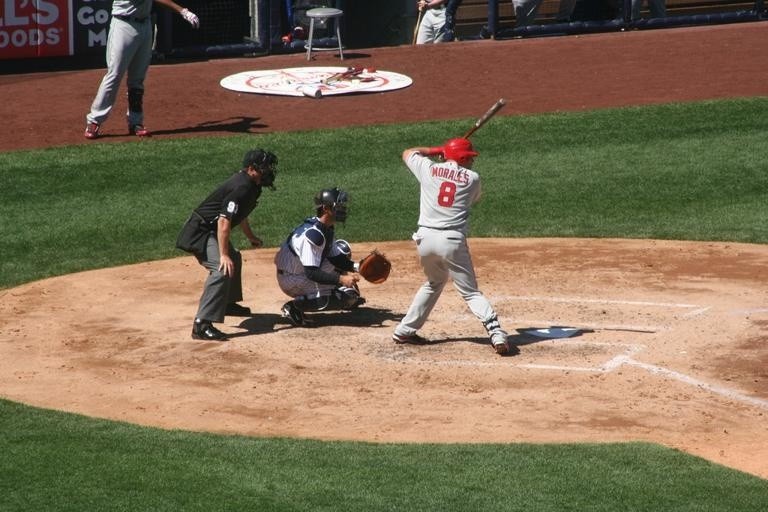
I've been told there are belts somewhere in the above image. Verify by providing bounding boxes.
[121,15,147,23]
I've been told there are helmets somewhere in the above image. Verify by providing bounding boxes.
[243,149,278,193]
[313,187,348,232]
[444,137,478,165]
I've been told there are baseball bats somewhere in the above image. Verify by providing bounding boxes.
[413,8,423,45]
[438,99,505,160]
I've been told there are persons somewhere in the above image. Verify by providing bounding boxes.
[416,0,457,44]
[391,137,510,354]
[275,189,392,327]
[174,150,278,342]
[83,0,200,138]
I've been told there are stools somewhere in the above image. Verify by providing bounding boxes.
[303,8,345,61]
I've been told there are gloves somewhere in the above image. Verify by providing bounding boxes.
[180,8,200,29]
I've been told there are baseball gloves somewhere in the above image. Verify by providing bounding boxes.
[357,248,391,285]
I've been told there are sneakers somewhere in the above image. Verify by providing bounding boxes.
[391,333,422,344]
[224,304,250,317]
[128,124,149,136]
[282,302,314,327]
[192,320,226,340]
[492,335,509,355]
[84,123,101,138]
